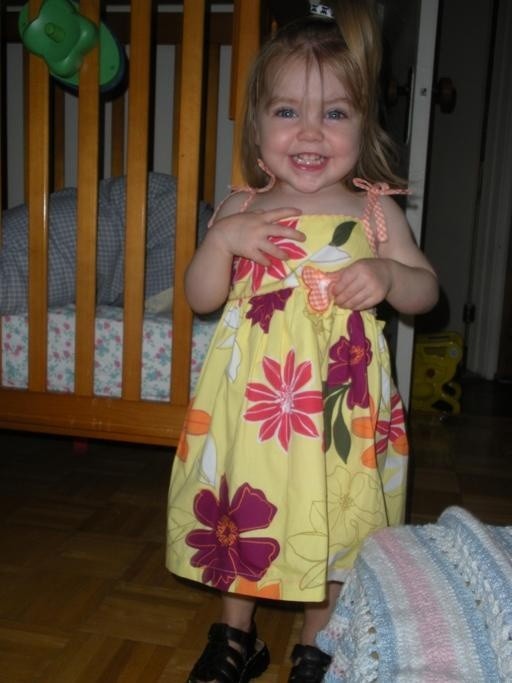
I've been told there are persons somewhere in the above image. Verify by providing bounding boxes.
[167,0,440,683]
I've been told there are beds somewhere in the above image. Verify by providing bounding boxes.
[1,3,259,448]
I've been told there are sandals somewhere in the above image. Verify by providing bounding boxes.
[186,621,269,683]
[287,644,331,683]
